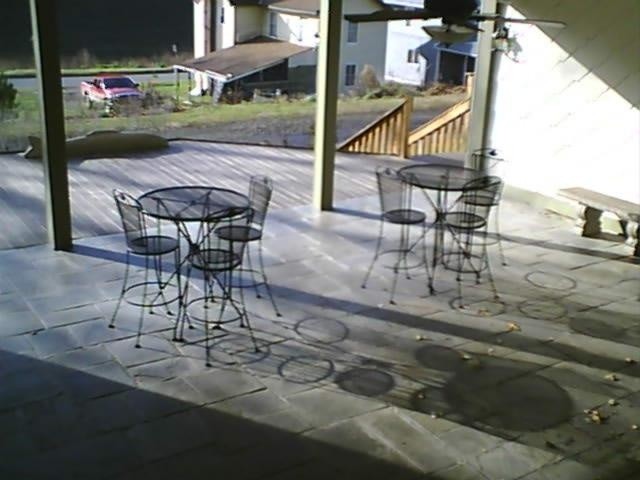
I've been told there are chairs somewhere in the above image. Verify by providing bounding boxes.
[110,174,282,367]
[359,148,506,309]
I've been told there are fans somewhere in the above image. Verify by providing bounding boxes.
[342,0,566,32]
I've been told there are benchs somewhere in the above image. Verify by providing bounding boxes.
[557,187,640,256]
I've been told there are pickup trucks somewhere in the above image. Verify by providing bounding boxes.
[80,71,147,110]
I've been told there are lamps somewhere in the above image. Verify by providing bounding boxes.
[422,14,476,47]
